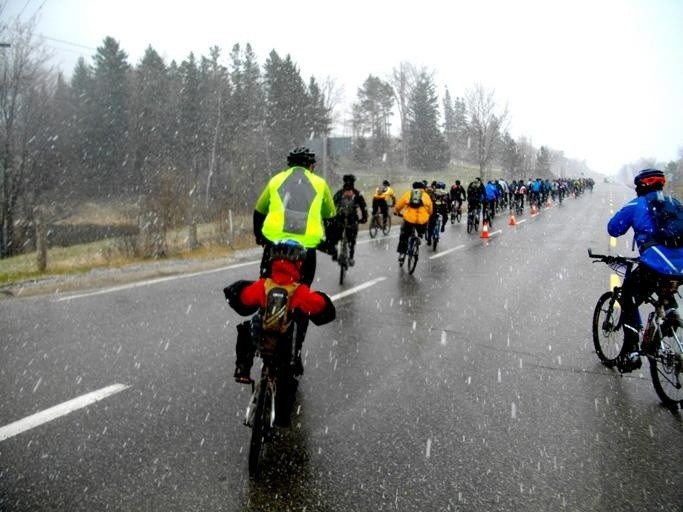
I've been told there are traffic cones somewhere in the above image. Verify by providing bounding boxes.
[479,221,491,239]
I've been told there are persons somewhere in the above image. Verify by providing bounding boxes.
[370,180,396,229]
[323,183,359,242]
[419,176,596,245]
[223,241,337,389]
[250,147,336,374]
[607,168,683,368]
[325,174,368,266]
[392,181,433,262]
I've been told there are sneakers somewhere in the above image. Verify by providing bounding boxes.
[617,352,641,370]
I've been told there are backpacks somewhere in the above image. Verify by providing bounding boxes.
[263,279,300,335]
[410,189,422,207]
[649,199,683,247]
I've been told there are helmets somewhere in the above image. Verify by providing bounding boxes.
[288,147,316,167]
[269,239,305,264]
[635,169,666,188]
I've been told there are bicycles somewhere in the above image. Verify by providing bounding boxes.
[420,199,444,250]
[235,346,304,476]
[393,210,422,274]
[369,202,393,237]
[584,245,683,411]
[447,183,594,231]
[323,215,363,283]
